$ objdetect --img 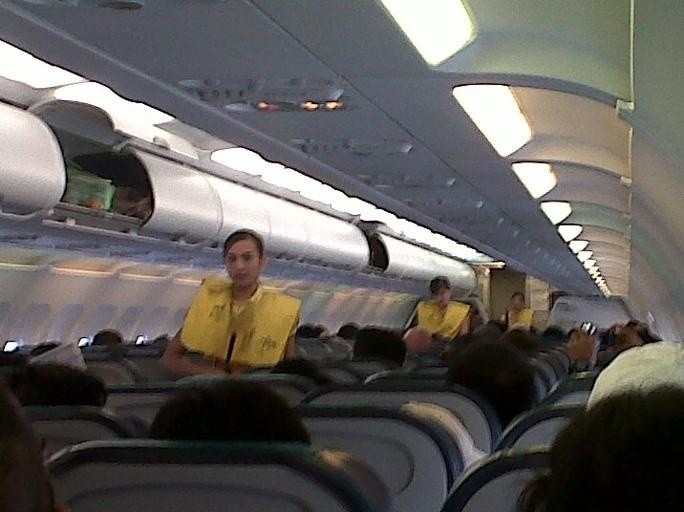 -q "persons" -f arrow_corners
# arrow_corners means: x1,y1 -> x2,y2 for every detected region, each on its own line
502,293 -> 536,333
162,228 -> 303,377
414,276 -> 471,341
1,317 -> 683,510
